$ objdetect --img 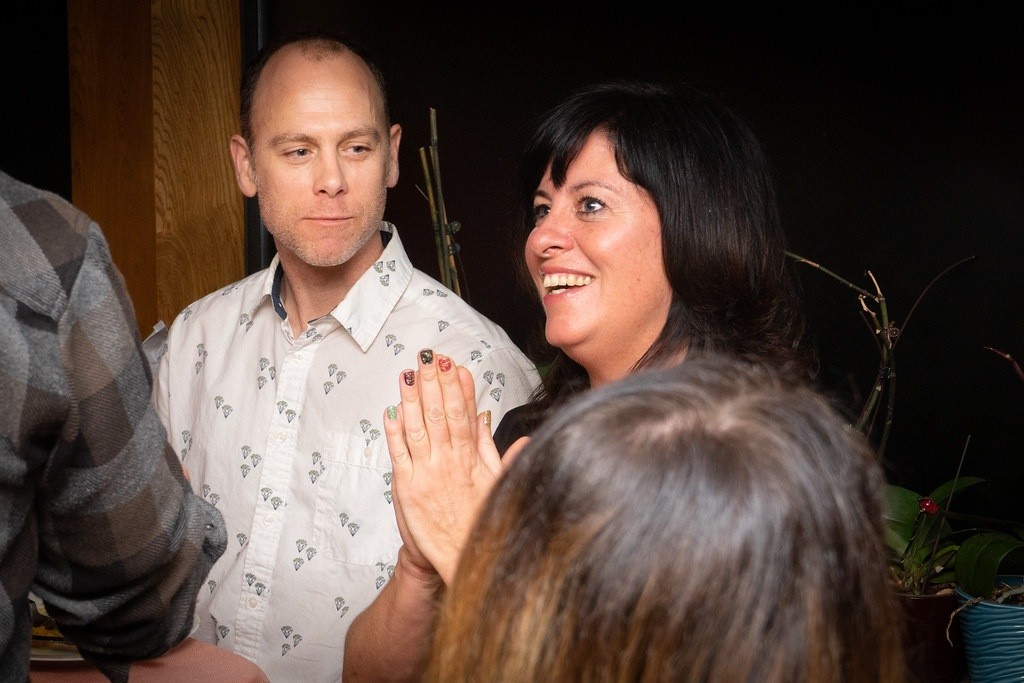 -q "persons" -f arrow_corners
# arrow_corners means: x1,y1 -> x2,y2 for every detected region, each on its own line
340,84 -> 820,682
0,167 -> 228,683
152,36 -> 547,681
428,360 -> 913,682
